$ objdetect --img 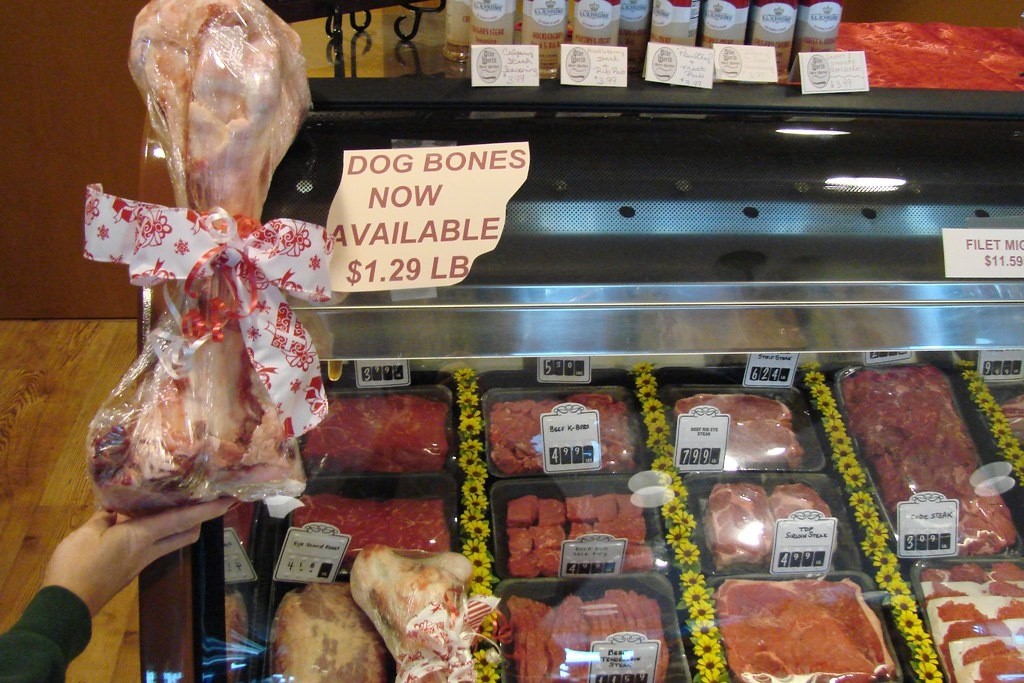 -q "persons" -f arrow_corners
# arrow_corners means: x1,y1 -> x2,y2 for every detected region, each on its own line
0,497 -> 242,683
